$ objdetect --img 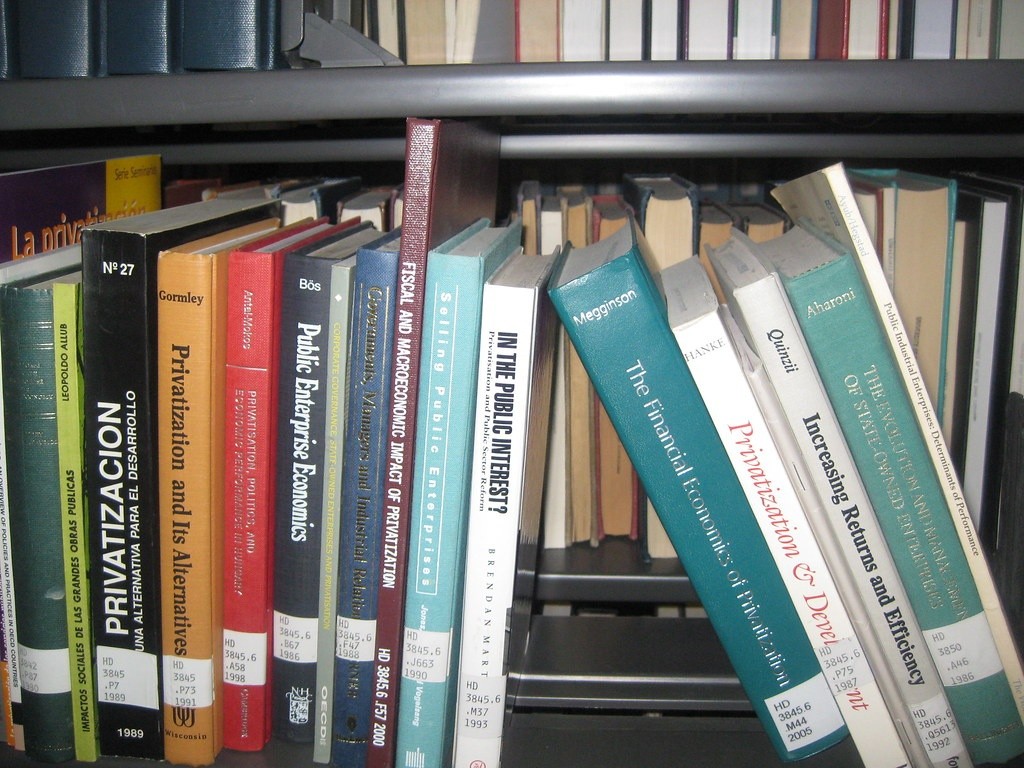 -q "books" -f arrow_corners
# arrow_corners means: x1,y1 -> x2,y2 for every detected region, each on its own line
0,152 -> 403,768
0,0 -> 1024,81
367,117 -> 1024,768
